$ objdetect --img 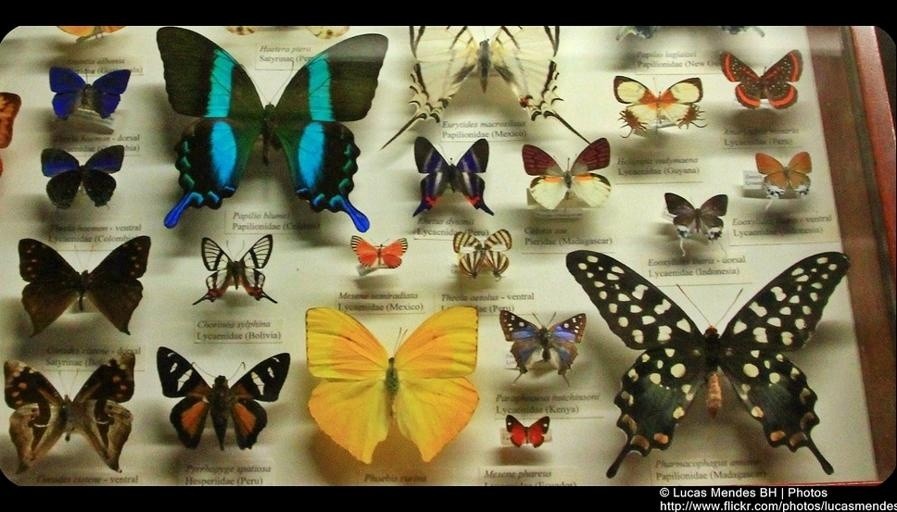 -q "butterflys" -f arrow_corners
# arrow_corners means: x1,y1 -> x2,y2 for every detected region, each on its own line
0,22 -> 855,486
